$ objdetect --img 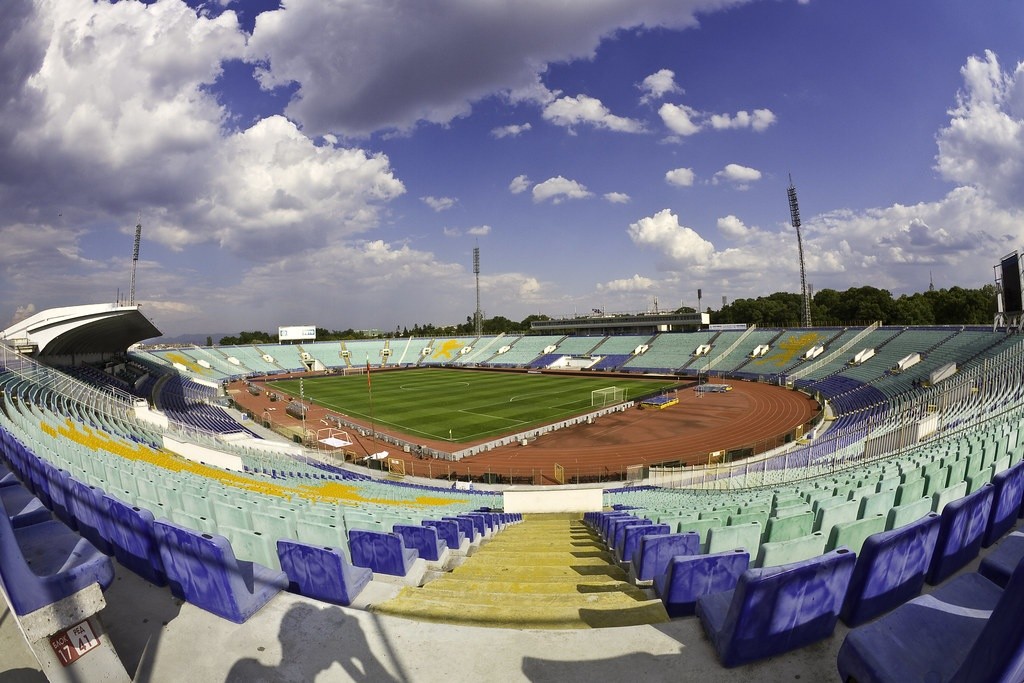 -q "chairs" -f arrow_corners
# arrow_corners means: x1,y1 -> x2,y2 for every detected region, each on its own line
0,327 -> 1024,683
